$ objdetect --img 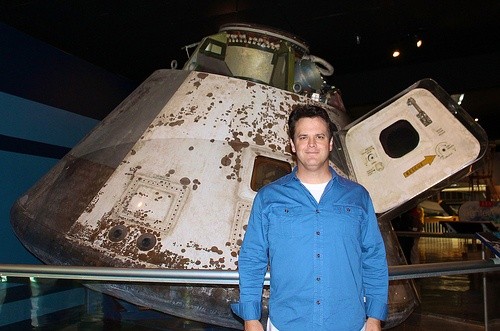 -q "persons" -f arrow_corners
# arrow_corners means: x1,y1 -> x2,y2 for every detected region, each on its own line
397,204 -> 426,264
231,105 -> 391,330
